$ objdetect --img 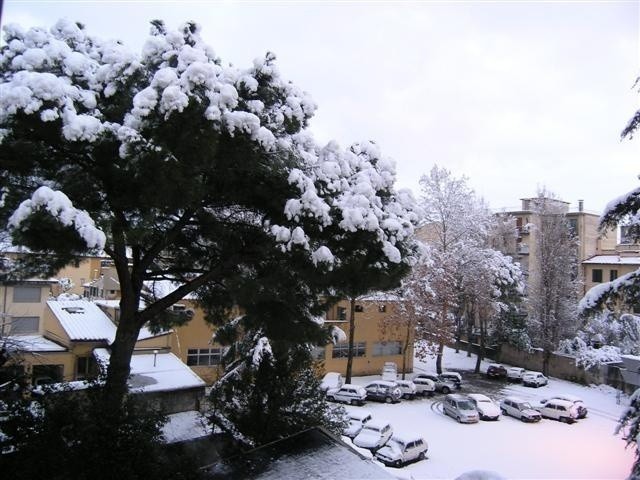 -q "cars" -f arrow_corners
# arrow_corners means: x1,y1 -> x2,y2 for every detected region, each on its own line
321,363 -> 590,470
